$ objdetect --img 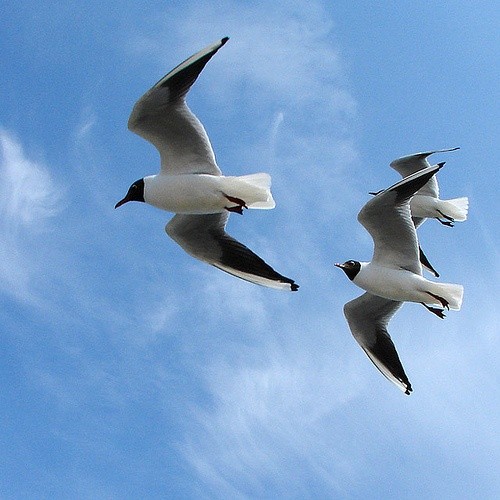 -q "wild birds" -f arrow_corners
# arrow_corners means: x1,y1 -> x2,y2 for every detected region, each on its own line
115,36 -> 300,292
334,146 -> 470,396
